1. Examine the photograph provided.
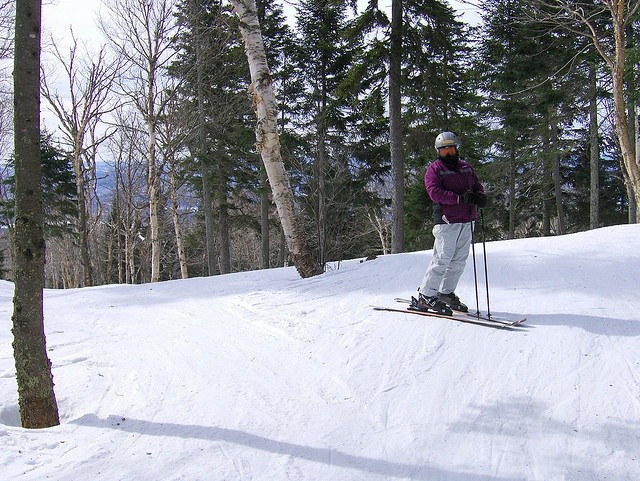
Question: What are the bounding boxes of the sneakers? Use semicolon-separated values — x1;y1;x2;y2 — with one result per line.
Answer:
437;292;467;312
418;294;452;316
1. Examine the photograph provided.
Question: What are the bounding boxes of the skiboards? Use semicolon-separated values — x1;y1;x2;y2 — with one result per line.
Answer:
373;298;526;328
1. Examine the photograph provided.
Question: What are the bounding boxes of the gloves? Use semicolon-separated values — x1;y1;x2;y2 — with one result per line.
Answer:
475;193;487;208
460;190;475;206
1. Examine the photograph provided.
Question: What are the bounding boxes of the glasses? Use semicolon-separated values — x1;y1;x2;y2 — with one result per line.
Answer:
439;146;457;156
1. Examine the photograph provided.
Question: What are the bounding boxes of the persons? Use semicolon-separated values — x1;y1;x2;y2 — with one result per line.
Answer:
417;132;487;316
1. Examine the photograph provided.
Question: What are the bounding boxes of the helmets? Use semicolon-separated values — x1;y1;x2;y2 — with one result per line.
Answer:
434;131;460;155
